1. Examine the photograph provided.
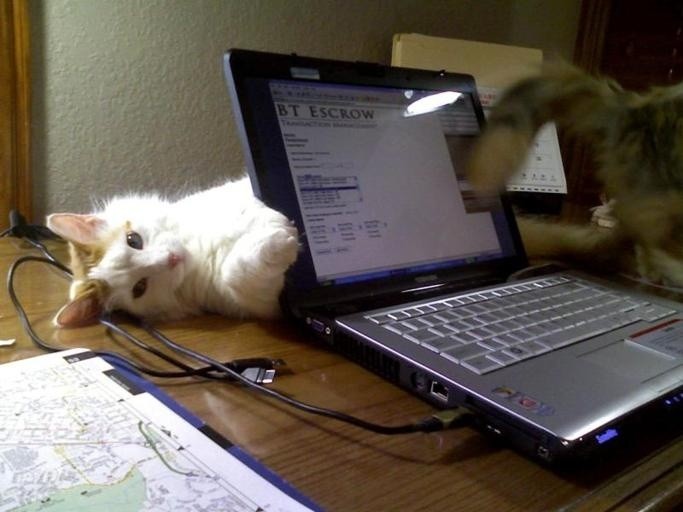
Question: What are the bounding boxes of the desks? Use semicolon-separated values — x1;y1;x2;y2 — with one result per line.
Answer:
0;224;683;512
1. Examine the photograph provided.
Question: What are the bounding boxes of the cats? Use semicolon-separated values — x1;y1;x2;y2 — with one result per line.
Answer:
466;66;683;288
45;172;614;329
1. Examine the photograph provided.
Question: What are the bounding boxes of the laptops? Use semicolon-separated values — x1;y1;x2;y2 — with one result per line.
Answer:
224;47;683;465
